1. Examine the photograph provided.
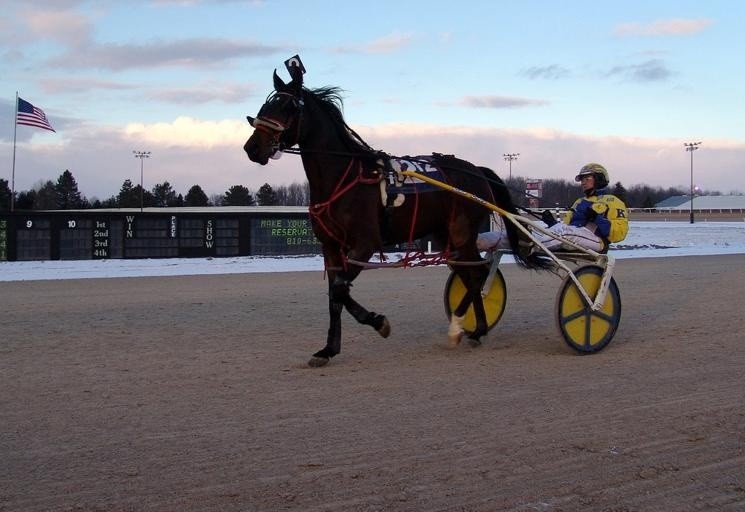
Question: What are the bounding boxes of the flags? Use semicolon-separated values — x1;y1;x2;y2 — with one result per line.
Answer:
16;97;56;133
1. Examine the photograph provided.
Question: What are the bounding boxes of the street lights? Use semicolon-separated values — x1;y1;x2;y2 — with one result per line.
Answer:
501;151;520;204
132;148;152;209
682;139;704;225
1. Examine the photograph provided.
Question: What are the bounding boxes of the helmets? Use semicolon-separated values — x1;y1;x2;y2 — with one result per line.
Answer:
574;161;609;183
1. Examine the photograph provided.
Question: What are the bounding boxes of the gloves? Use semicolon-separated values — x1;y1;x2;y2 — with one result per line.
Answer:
542;209;554;224
584;207;597;221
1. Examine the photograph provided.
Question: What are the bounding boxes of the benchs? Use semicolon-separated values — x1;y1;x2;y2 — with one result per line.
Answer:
493;248;607;257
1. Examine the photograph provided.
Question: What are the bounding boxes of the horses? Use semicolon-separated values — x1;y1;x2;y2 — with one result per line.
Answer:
243;65;566;368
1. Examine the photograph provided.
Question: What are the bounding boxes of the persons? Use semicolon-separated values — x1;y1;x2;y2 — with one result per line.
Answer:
476;162;630;258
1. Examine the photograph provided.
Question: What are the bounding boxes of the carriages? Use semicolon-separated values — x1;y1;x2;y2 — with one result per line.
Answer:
238;62;624;370
